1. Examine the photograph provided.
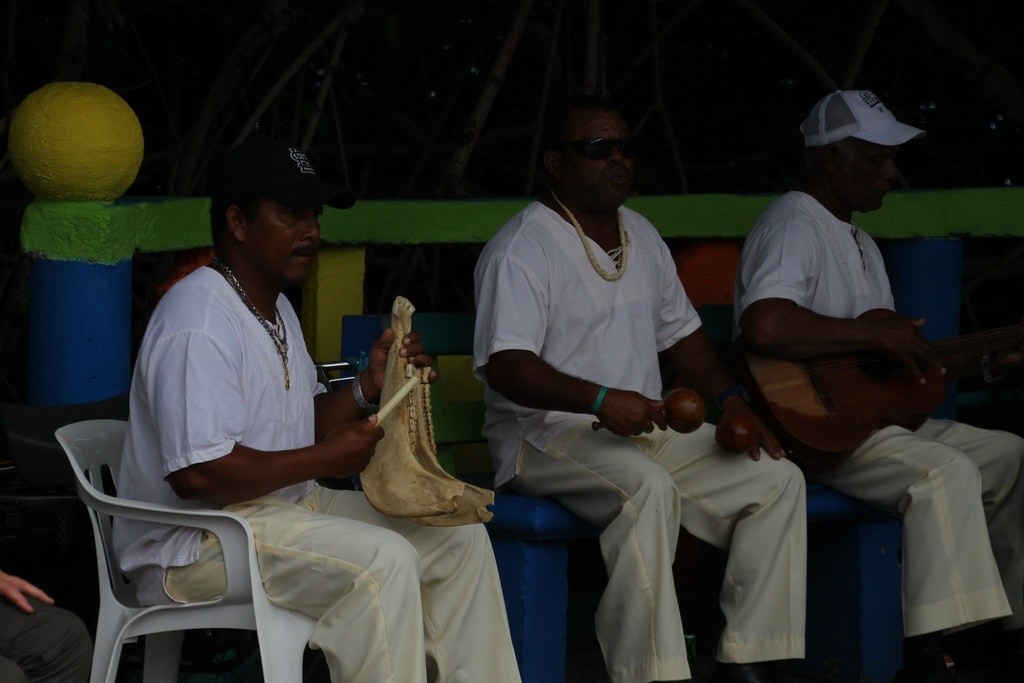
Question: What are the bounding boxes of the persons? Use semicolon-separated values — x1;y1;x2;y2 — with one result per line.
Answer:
473;97;806;683
112;139;523;683
732;91;1024;683
0;569;94;683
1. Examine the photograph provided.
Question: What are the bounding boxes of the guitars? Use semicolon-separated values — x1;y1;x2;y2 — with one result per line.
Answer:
732;298;1024;470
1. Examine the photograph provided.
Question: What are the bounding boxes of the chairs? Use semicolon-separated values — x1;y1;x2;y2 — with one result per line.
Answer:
54;418;320;683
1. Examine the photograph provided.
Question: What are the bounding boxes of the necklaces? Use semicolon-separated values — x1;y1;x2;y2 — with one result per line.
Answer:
210;253;291;395
549;186;627;280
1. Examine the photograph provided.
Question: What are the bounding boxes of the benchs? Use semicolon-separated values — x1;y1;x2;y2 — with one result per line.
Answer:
456;471;906;683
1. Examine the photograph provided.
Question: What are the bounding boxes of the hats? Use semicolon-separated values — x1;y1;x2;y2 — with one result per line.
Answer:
801;90;926;147
210;139;327;210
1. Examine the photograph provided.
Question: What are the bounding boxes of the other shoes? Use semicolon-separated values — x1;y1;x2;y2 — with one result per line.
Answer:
709;661;785;683
892;636;1024;683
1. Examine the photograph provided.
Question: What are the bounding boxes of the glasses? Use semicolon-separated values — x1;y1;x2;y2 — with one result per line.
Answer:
546;136;635;160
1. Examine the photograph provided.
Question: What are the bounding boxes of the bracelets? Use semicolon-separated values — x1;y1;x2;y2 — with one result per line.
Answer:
352;373;380;413
591;386;608;416
714;388;742;410
